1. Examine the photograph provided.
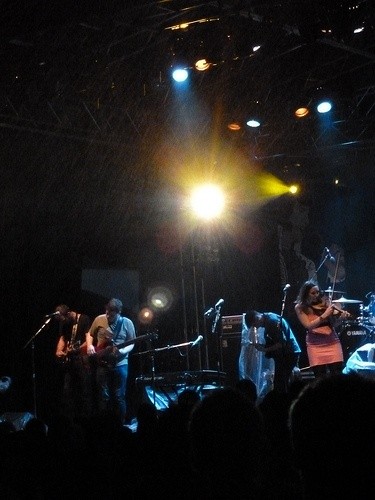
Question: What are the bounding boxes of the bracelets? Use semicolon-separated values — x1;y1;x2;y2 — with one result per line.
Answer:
268;344;273;352
319;316;326;321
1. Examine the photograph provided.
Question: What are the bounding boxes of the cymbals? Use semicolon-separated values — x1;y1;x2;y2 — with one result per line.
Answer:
320;290;344;293
331;299;365;305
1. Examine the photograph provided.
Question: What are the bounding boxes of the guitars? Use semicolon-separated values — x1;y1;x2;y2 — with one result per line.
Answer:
60;341;80;368
238;339;276;360
78;330;159;370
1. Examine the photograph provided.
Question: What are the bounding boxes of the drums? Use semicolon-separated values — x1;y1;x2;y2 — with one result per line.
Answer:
341;320;368;358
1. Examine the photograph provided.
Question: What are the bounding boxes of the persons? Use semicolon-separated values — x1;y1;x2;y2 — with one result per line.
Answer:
322;243;349;310
53;298;136;438
294;280;351;380
245;309;301;393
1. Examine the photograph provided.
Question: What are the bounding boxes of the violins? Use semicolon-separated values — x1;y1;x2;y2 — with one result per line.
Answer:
313;294;353;318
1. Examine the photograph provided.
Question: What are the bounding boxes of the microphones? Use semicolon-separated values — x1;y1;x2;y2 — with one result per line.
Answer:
214;299;224;307
45;311;61;318
204;307;214;316
283;284;291;291
325;246;337;264
191;335;203;347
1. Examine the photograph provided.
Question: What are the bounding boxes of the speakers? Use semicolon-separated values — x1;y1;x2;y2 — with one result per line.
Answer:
219;332;242;375
135;375;195;401
339;324;368;358
